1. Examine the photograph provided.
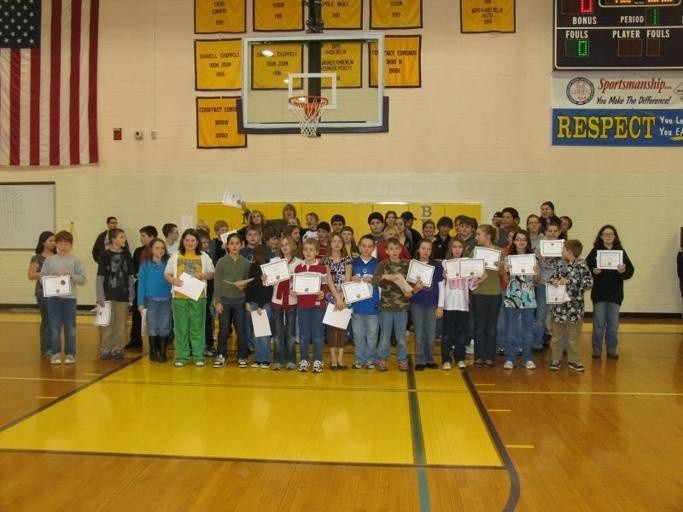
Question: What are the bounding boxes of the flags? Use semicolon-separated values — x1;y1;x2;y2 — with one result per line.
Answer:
0;0;100;167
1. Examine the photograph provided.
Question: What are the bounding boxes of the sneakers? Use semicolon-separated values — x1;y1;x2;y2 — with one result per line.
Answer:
607;353;619;359
102;352;113;359
503;361;512;369
549;360;560;370
45;350;76;363
526;360;536;369
568;361;584;370
115;350;123;359
399;360;408;370
415;359;493;371
592;352;601;357
175;348;323;372
354;361;386;371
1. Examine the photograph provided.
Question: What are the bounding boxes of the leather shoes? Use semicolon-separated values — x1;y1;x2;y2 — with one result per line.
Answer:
330;361;346;369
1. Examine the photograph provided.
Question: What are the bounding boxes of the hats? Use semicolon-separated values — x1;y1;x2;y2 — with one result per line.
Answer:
401;212;417;219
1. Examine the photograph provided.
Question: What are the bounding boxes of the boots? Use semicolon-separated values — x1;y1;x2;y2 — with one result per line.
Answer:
149;337;167;362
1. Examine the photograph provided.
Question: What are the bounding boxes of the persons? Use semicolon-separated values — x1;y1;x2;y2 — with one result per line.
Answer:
585;225;634;360
92;202;593;372
28;231;57;358
39;231;87;365
